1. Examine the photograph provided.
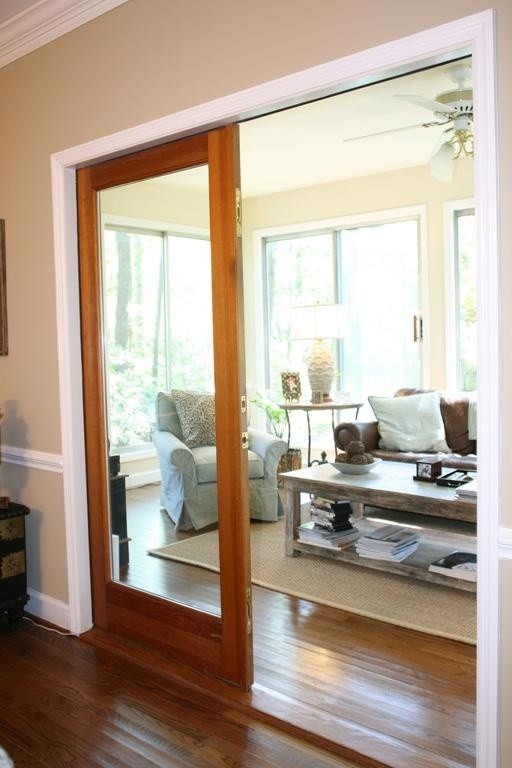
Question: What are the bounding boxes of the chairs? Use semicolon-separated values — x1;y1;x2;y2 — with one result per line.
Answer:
153;389;287;532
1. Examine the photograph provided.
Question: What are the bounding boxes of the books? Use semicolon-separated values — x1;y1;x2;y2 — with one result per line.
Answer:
455;479;478;498
428;551;477;582
295;495;419;563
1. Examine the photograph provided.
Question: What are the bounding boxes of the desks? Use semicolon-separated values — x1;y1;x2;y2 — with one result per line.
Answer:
110;474;132;565
0;501;30;625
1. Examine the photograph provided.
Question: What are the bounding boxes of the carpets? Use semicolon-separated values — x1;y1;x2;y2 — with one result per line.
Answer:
144;501;477;648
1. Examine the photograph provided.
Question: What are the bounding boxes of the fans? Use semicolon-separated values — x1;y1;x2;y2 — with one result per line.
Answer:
342;64;474;182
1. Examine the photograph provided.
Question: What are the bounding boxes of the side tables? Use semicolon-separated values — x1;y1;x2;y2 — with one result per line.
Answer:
279;400;364;467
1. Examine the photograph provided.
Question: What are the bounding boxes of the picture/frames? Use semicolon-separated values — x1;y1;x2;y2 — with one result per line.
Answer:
282;371;301;403
1;218;9;356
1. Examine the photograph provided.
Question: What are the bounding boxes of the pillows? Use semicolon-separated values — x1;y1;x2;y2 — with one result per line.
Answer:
171;390;217;447
367;393;448;452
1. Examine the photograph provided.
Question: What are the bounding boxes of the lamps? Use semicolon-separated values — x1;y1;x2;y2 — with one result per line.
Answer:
292;300;351;404
430;118;475;182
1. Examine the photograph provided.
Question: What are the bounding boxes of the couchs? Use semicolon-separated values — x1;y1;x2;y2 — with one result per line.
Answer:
333;386;476;472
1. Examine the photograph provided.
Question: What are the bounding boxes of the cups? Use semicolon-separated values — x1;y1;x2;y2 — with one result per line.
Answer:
311;389;324;404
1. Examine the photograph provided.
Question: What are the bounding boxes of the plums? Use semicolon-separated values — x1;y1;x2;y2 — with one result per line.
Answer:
335;440;374;465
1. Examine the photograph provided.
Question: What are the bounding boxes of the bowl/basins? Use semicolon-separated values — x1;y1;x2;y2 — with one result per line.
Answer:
328;457;384;473
